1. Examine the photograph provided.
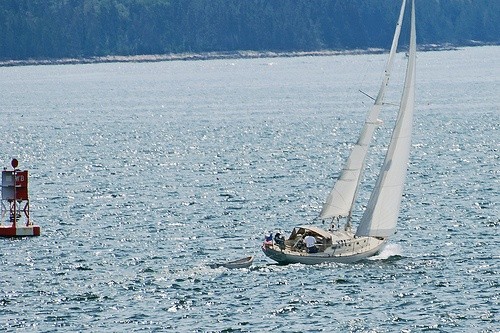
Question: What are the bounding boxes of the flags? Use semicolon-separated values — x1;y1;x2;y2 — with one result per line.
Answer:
264;234;273;246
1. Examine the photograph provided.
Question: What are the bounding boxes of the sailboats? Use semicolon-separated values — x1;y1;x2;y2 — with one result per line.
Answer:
262;0;417;265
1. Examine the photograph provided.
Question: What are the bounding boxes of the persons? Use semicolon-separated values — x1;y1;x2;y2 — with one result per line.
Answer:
303;231;319;253
